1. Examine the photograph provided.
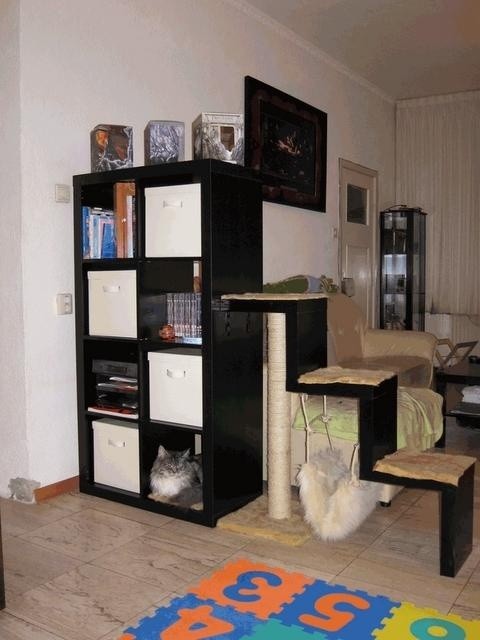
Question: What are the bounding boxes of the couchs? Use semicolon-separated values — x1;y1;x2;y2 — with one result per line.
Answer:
260;288;451;506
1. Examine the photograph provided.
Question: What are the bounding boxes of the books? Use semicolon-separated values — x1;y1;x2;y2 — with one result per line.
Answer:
87;376;139;420
167;261;202;345
82;179;136;259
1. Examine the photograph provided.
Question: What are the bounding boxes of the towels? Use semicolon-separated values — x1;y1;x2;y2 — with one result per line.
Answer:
370;446;478;489
296;362;398;386
217;290;329;302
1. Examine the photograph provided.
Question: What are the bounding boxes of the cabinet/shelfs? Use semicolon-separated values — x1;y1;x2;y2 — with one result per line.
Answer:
71;155;268;529
378;208;427;333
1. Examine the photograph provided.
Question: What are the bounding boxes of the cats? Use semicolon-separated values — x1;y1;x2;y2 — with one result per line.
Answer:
146;443;203;506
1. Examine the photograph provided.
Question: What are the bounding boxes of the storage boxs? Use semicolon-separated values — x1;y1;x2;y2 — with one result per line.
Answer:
142;183;203;260
192;111;247;166
86;270;140;340
147;345;206;430
92;414;141;495
88;124;134;173
144;118;187;165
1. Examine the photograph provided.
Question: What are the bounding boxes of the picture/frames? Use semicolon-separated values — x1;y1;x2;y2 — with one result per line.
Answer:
242;76;329;214
